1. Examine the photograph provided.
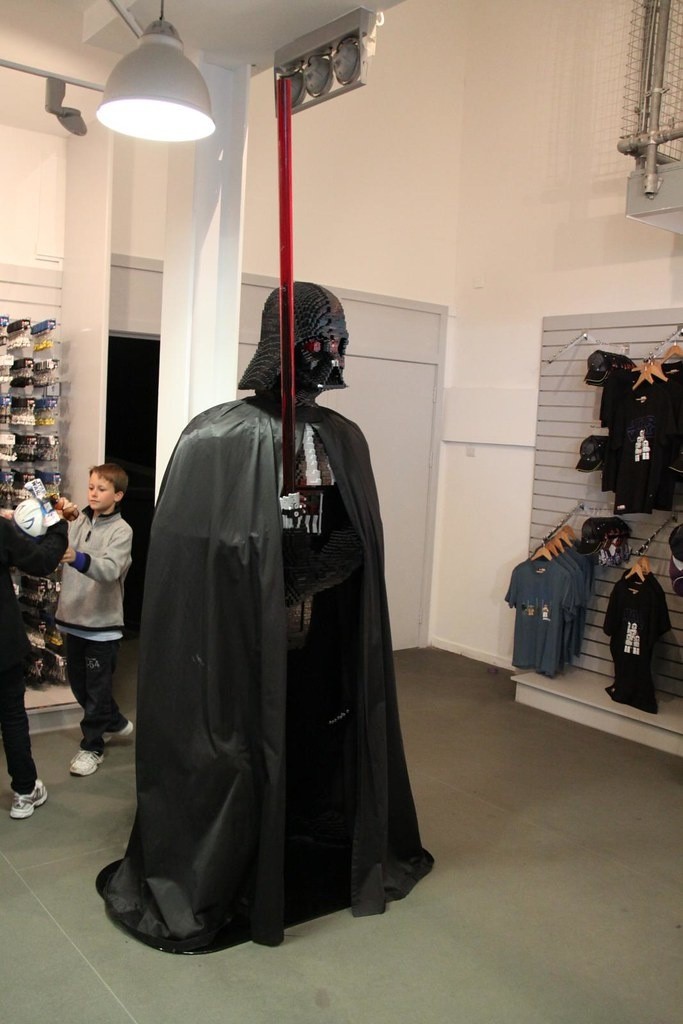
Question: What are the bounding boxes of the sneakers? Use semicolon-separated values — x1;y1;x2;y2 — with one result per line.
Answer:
10;779;48;818
69;749;104;776
101;720;133;741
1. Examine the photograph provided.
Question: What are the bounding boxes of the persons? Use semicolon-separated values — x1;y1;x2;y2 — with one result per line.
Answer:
61;465;134;775
96;283;435;956
0;498;69;819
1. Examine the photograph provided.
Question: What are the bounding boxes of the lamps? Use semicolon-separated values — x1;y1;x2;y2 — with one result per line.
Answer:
274;7;385;118
96;0;217;143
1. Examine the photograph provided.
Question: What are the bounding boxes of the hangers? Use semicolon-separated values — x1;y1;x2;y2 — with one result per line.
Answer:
632;334;683;401
625;552;645;593
637;544;651;575
531;517;576;573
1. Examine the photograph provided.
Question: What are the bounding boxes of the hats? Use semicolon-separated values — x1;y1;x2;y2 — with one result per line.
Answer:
584;350;637;387
668;523;683;571
575;435;608;473
574;517;632;555
